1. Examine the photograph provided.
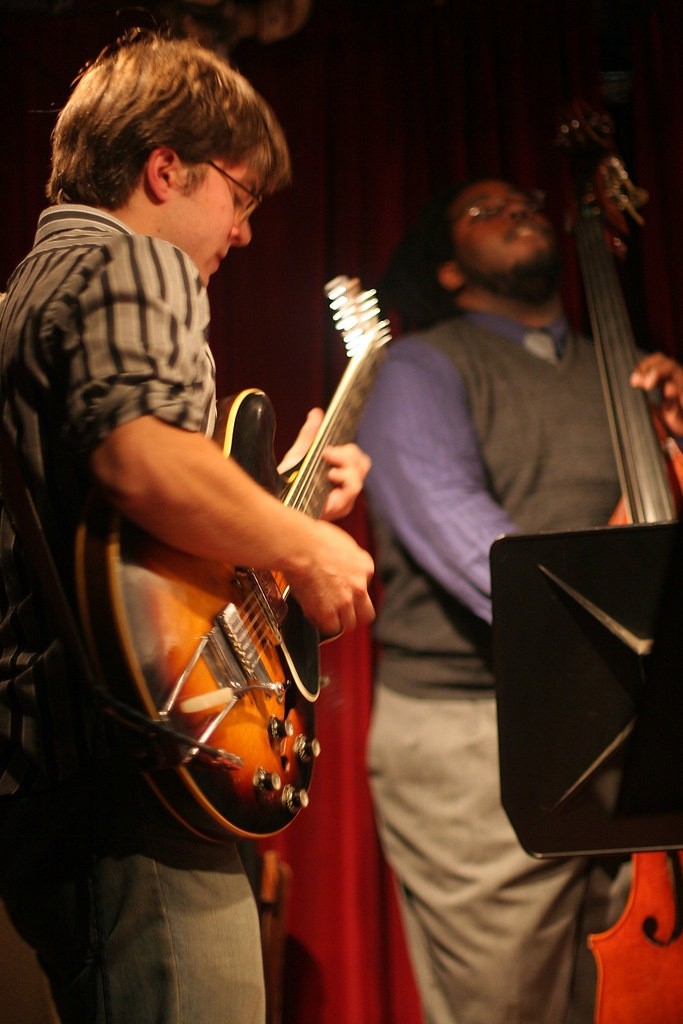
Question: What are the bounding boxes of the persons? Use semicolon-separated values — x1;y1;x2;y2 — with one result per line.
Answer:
0;27;375;1024
355;176;683;1024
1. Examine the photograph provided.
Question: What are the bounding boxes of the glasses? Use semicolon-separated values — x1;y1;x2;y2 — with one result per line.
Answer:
206;159;262;223
450;195;539;251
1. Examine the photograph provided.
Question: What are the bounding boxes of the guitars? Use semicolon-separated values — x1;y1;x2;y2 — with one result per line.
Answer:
77;271;393;848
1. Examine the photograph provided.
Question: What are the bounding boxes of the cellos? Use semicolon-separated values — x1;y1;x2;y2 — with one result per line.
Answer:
557;117;683;1024
